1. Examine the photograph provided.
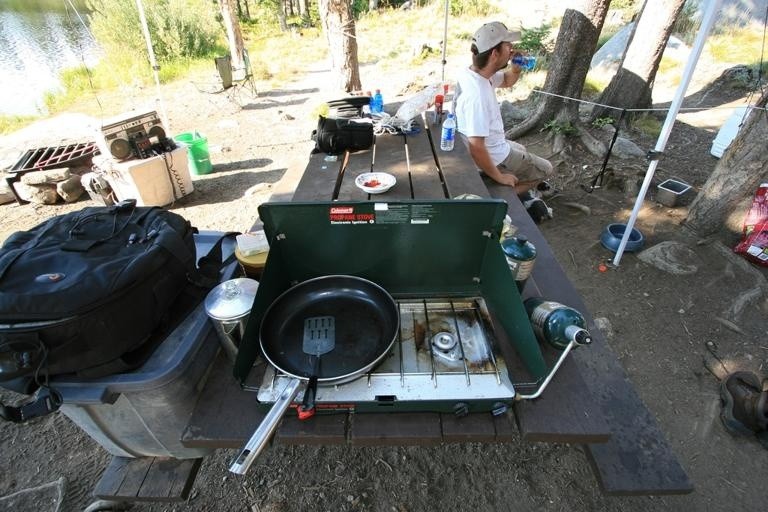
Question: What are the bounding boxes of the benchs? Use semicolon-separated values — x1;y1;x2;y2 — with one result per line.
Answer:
481;175;697;496
90;456;203;504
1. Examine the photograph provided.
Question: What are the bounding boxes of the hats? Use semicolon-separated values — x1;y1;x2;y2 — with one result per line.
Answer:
471;21;522;53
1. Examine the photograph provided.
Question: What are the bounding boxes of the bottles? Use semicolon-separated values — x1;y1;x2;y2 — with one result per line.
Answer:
441;113;455;151
366;89;384;115
443;84;449;102
509;54;536;71
434;95;443;124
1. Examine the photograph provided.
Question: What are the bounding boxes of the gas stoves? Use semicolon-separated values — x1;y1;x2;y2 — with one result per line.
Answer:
253;292;517;411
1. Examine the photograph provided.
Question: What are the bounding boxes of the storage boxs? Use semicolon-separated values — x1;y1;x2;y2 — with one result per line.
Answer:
90;139;194;208
31;226;245;463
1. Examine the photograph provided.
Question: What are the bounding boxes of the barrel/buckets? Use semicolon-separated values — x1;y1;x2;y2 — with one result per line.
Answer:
177;133;213;175
234;245;269;279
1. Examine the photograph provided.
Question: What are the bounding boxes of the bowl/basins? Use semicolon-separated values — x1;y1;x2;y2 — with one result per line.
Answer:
599;222;643;252
355;171;397;194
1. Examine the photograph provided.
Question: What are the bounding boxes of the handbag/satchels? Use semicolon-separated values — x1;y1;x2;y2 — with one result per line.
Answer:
311;114;373;156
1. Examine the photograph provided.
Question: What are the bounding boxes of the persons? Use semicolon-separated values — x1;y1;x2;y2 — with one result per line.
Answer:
449;21;554;195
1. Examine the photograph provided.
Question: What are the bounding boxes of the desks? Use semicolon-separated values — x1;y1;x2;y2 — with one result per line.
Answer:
177;102;615;451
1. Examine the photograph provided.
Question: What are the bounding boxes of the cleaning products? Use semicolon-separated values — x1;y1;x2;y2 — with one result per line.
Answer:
375;90;383;111
367;92;374;113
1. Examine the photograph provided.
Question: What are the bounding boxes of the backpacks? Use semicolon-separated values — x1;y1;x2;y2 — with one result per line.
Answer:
0;199;222;423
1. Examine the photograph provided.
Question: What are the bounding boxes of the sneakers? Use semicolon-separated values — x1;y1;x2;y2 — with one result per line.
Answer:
720;370;768;437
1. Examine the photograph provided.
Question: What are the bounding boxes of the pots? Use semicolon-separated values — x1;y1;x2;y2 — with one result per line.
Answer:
227;275;399;478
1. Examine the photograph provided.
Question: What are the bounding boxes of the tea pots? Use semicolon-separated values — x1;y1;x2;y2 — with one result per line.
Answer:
203;277;261;369
